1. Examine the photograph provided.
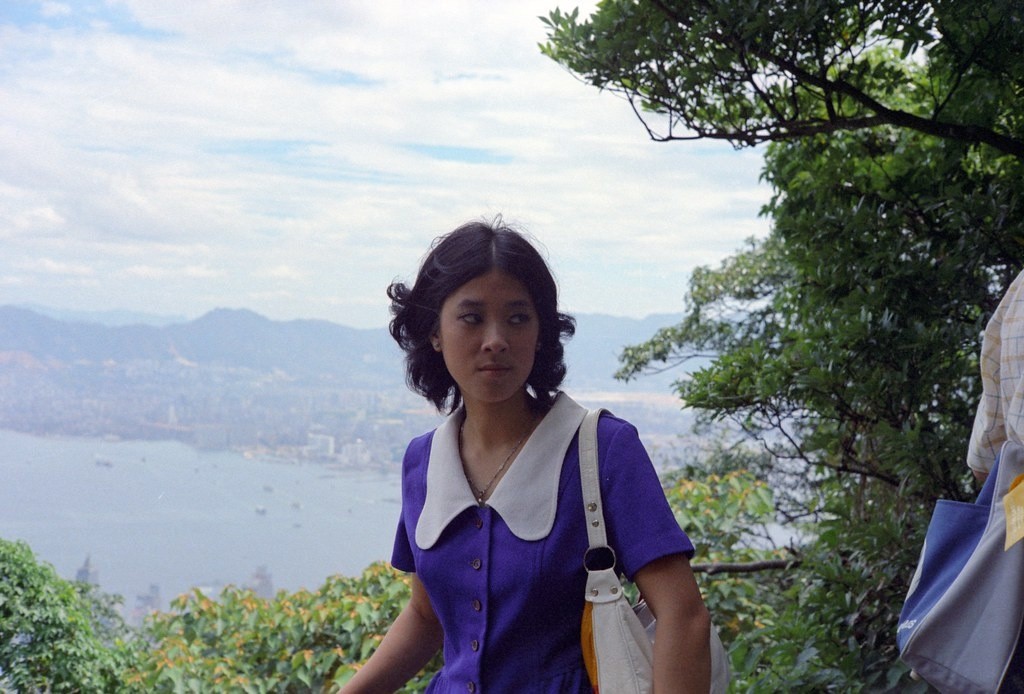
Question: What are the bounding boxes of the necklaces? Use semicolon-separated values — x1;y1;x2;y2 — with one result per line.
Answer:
457;419;537;501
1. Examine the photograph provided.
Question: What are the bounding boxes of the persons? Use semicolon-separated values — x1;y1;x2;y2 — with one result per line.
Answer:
891;266;1024;694
330;219;714;694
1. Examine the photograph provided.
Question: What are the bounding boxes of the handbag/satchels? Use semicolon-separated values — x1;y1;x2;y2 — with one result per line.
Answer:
579;409;731;694
896;439;1024;694
